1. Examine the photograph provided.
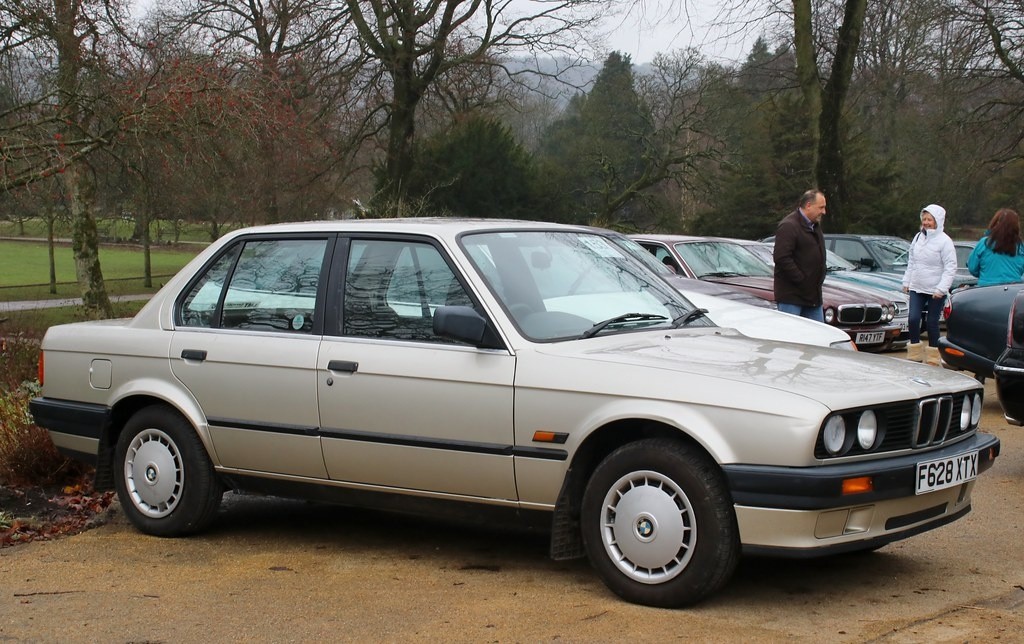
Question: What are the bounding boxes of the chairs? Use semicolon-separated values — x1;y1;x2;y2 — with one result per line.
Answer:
447;274;509;307
345;271;400;339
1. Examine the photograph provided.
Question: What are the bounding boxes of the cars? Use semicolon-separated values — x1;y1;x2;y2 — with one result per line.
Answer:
625;233;904;345
186;236;860;350
755;234;982;294
765;242;950;334
937;279;1024;379
26;212;1002;610
993;282;1024;428
577;224;777;309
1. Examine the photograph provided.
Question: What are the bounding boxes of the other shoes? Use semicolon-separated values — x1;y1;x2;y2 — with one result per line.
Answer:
973;374;985;384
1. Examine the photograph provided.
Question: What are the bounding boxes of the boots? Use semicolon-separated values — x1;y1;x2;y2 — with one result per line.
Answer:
906;340;923;363
924;346;941;366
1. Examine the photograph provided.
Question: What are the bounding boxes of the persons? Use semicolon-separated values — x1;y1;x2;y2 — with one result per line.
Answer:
966;207;1024;384
902;203;959;369
771;188;827;325
